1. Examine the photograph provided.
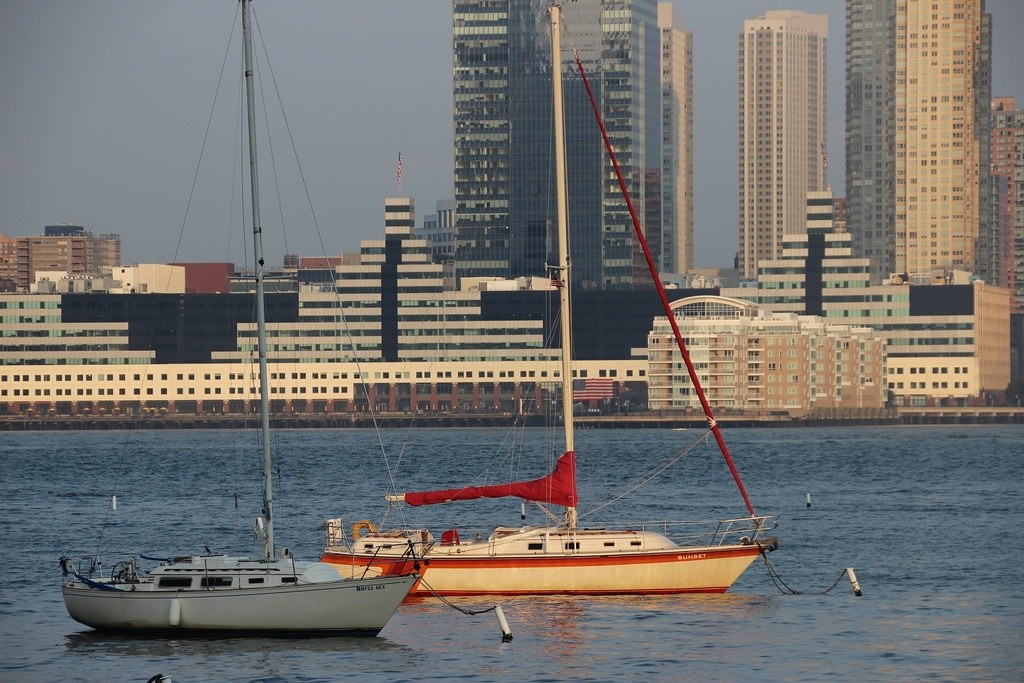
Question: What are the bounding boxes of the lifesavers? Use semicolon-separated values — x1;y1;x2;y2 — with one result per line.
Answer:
351;520;377;541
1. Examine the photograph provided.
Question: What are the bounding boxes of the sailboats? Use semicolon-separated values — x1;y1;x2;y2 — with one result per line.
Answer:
59;0;418;636
319;0;778;600
